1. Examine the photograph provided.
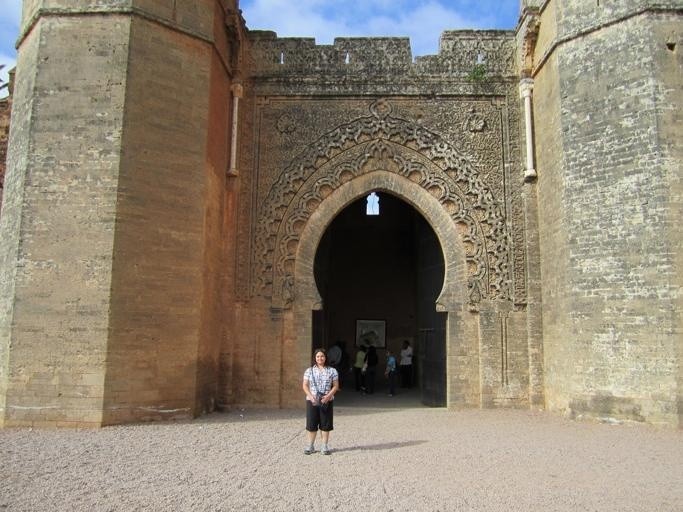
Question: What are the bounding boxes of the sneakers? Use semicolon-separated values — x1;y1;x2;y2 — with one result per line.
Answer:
304;446;315;455
320;446;329;456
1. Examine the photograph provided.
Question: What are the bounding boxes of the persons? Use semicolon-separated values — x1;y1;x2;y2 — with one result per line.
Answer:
326;343;342;369
302;347;339;455
353;344;366;391
360;345;378;394
383;349;397;397
398;340;413;393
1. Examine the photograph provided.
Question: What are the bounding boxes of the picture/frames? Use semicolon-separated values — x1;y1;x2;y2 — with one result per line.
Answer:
355;318;387;349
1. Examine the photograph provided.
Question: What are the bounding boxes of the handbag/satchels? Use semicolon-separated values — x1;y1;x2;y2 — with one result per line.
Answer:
314;392;329;406
